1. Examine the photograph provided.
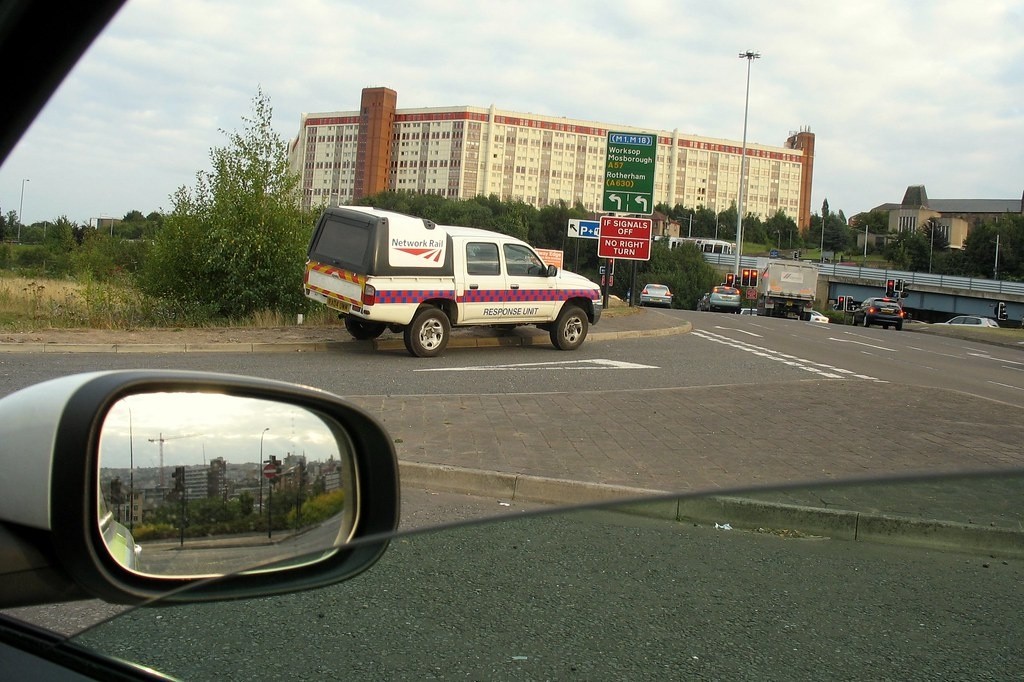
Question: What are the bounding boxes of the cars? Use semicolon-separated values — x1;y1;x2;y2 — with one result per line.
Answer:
697;285;757;315
934;315;1001;329
850;296;903;331
640;283;674;309
810;310;829;323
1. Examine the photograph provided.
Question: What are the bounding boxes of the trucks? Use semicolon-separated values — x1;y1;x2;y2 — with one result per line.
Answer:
757;260;817;320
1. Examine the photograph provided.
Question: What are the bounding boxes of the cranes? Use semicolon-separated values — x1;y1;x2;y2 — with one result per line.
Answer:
148;432;205;487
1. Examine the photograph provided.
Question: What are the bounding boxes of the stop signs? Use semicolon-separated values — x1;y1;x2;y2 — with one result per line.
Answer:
263;464;277;479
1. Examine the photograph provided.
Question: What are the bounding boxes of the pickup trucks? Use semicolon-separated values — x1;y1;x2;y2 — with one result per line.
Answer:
303;203;603;359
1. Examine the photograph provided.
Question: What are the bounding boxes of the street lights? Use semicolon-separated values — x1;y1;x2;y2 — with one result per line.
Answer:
989;234;1002;278
733;47;762;276
260;427;270;517
927;219;934;274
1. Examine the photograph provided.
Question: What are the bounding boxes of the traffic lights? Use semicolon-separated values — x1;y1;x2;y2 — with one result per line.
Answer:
749;269;759;288
740;269;750;286
996;302;1008;321
610;258;615;275
833;296;846;311
895;278;903;292
269;455;282;484
845;296;854;311
172;466;184;491
721;273;735;288
900;281;910;299
732;273;742;288
885;279;896;297
792;248;802;259
